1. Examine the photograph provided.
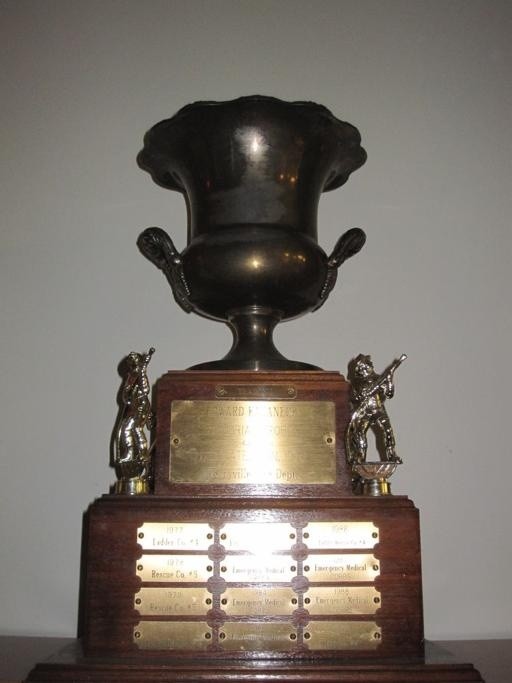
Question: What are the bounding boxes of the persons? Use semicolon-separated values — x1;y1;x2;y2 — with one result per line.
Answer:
346;352;401;464
108;348;158;460
